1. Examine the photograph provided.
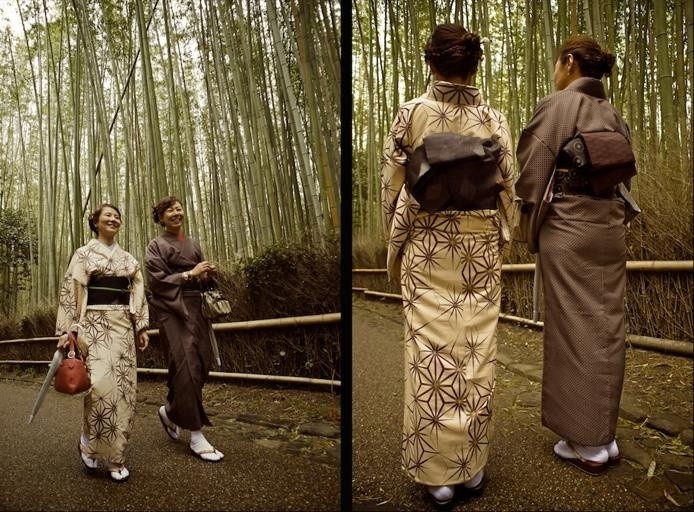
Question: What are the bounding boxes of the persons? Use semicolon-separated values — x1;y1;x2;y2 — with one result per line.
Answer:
515;32;643;478
379;23;522;510
142;197;230;463
52;203;153;483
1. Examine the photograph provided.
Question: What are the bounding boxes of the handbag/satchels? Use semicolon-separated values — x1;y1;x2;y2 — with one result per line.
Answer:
52;358;89;393
202;291;231;319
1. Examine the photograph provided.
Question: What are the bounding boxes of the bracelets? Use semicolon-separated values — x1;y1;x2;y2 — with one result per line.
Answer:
188;270;192;280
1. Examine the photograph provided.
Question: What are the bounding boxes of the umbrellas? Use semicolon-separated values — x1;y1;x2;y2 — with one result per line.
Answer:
532;253;541;323
27;323;84;424
206;319;221;366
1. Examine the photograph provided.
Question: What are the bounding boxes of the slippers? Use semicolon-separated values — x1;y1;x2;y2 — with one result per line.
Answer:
434;494;455;509
469;478;486;498
78;443;98;471
190;444;224;463
158;406;180;441
553;438;622;475
106;462;129;482
